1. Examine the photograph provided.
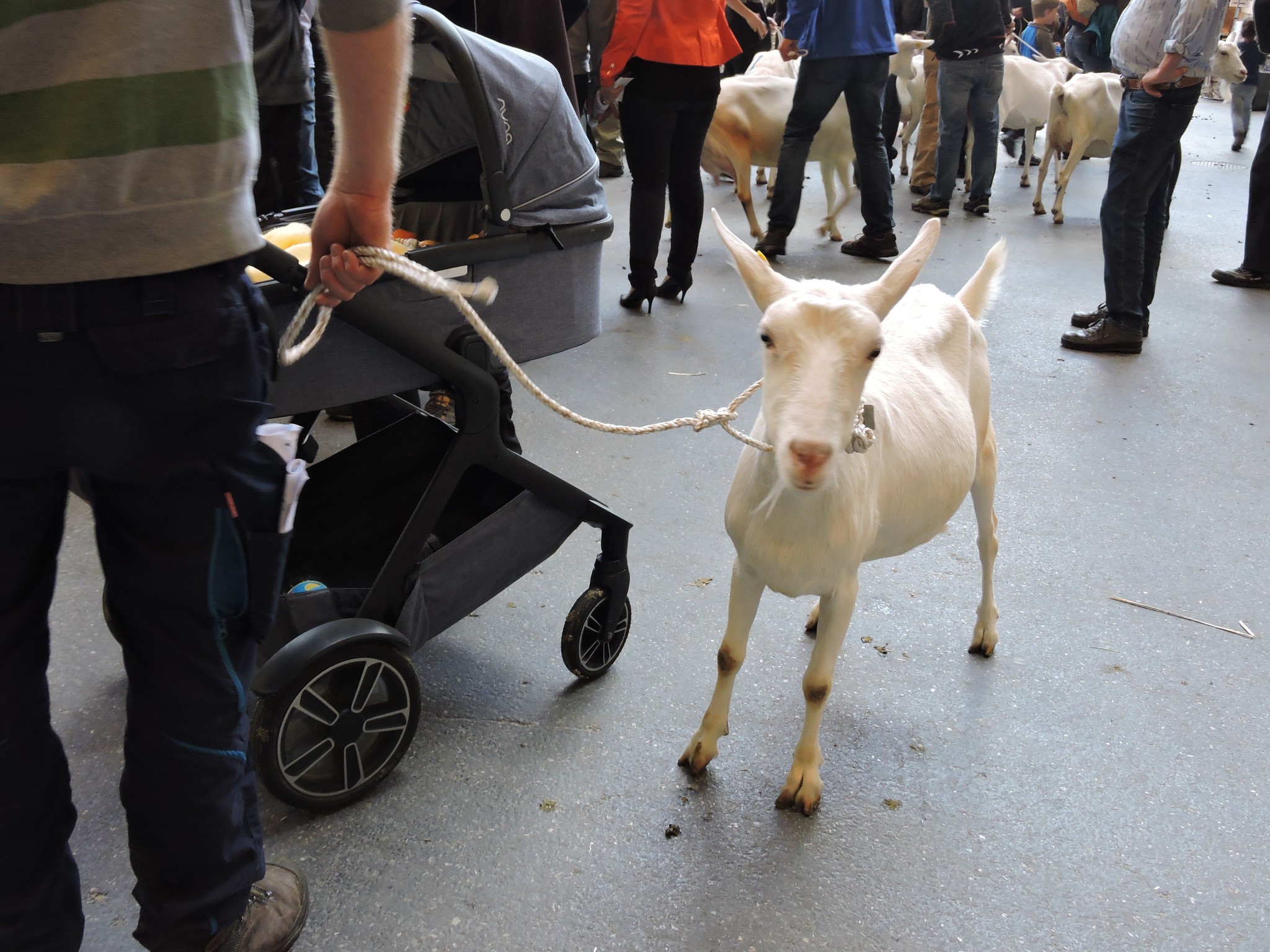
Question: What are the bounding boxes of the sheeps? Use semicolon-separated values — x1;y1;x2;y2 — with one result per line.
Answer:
670;203;1013;822
698;28;1252;242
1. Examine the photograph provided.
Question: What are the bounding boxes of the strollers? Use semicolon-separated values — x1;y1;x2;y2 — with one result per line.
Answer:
235;1;633;810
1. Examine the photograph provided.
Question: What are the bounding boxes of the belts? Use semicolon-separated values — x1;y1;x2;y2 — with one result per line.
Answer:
1117;74;1205;91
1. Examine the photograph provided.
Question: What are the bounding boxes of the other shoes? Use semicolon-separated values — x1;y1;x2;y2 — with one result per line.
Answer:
1060;318;1142;354
1212;267;1270;287
1018;154;1043;166
1001;133;1016;157
911;199;950;217
963;197;990;212
598;160;624;178
1070;302;1149;337
1061;151;1091;160
1232;132;1246;151
910;184;935;193
208;855;310;952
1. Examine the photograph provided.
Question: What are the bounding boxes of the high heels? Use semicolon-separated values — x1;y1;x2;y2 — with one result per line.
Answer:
619;284;656;314
656;271;692;304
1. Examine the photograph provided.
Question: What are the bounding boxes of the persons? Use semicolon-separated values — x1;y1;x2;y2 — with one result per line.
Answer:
558;0;624;179
1062;0;1230;354
717;0;788;182
1211;0;1270;289
1231;17;1267;152
0;0;411;952
852;0;1130;217
600;0;743;314
753;0;899;257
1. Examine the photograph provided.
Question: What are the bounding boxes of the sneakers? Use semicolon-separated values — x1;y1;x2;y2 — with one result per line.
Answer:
754;229;788;256
842;232;898;256
